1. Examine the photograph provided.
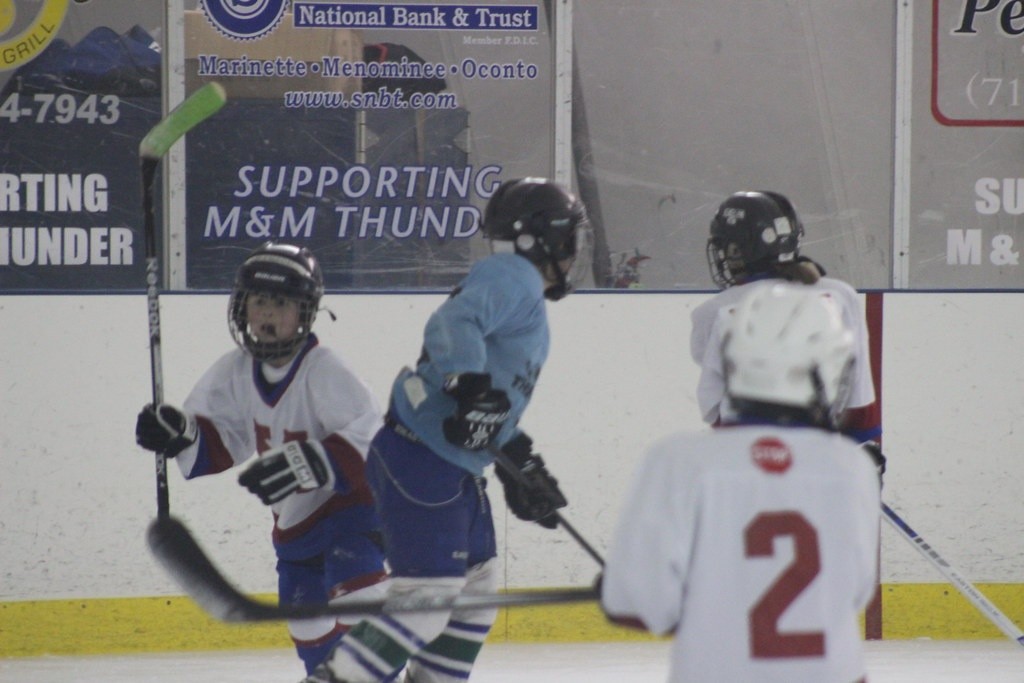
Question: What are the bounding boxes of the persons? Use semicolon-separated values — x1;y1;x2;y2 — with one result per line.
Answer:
590;284;883;683
688;189;875;440
135;241;383;681
297;175;589;683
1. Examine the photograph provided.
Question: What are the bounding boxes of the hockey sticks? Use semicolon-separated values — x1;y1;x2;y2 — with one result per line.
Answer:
133;78;608;625
879;504;1024;645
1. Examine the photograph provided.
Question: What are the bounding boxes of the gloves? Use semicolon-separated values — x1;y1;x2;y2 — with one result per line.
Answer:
442;372;512;451
495;432;567;530
134;403;199;459
238;440;328;506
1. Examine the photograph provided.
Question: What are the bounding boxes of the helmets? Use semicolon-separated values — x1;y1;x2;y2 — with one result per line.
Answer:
482;177;595;302
719;283;861;429
227;240;337;363
706;190;805;290
